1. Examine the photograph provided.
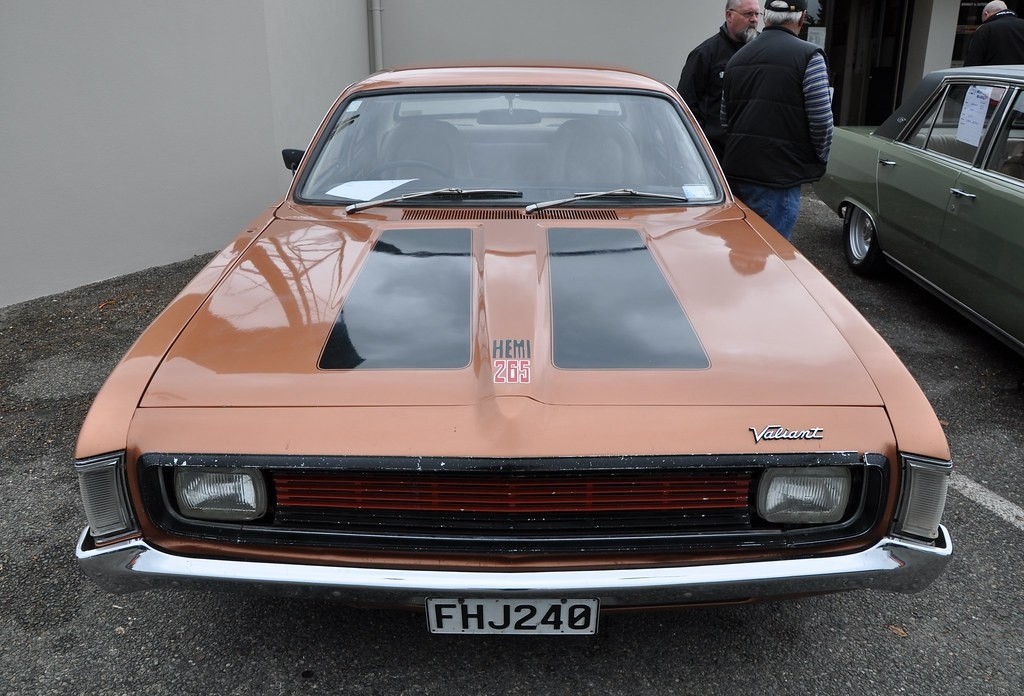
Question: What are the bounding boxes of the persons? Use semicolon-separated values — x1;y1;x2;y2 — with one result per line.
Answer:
676;0;761;165
962;0;1024;119
721;0;834;241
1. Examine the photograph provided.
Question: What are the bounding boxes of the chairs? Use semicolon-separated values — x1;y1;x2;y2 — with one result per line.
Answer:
549;118;643;186
378;120;469;188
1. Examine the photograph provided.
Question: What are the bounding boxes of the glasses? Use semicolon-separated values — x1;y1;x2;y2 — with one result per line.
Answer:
729;8;763;19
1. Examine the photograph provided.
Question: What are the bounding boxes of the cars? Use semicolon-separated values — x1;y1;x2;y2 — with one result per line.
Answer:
816;64;1024;360
74;65;954;637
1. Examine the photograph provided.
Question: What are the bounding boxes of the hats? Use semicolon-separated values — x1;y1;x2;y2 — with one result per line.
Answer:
764;0;807;13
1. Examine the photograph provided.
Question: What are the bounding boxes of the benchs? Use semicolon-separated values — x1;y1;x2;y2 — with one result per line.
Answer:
918;133;1024;162
